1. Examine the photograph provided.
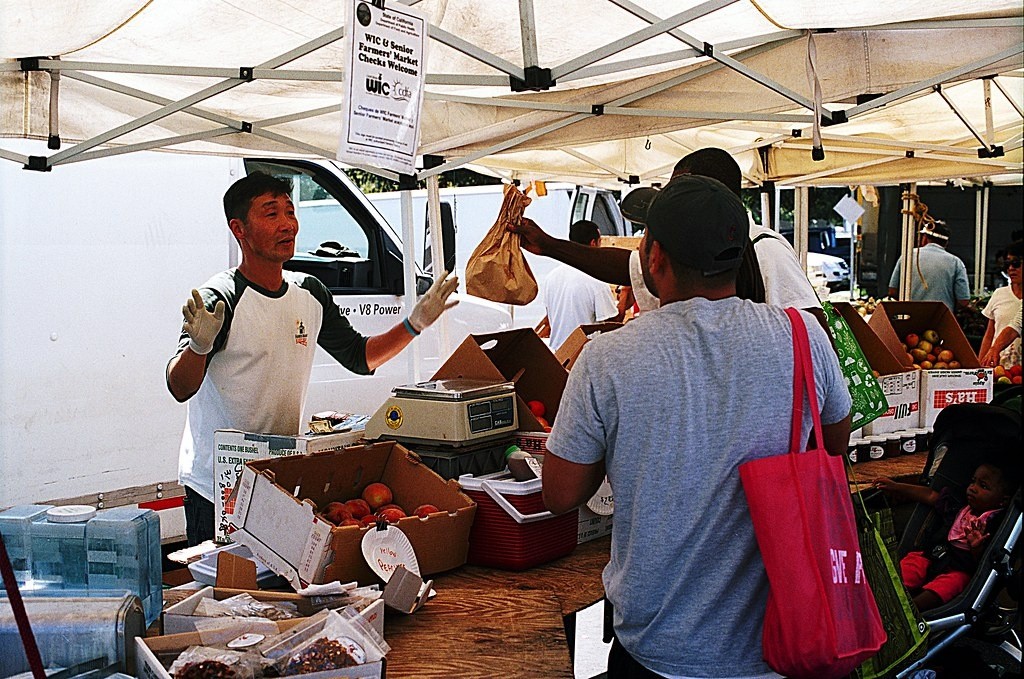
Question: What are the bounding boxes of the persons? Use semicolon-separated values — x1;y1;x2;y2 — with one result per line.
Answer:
889;223;970;311
982;306;1023;367
165;170;461;545
978;227;1024;365
544;220;631;349
506;148;838;360
873;458;1015;611
543;174;853;679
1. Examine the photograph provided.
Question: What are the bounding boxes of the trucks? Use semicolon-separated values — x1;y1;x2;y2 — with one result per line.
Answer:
297;183;633;306
0;137;512;510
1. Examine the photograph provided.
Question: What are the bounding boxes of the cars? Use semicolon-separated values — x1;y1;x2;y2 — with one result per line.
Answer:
807;252;849;286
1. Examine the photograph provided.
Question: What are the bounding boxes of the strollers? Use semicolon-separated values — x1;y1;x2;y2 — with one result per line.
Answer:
888;384;1024;679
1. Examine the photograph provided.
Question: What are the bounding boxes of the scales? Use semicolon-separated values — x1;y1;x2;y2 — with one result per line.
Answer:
364;378;519;448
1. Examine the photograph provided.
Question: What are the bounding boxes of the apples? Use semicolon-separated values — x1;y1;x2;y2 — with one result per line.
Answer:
529;400;552;433
985;364;1022;384
871;370;880;378
902;330;960;368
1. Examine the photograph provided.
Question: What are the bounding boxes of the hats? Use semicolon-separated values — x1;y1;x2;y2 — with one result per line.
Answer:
917;219;950;241
620;174;749;274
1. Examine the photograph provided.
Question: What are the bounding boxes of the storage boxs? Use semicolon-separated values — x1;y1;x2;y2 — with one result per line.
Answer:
832;301;904;376
134;585;387;679
516;430;615;545
429;328;570;433
862;366;921;437
919;367;994;428
458;465;579;570
228;440;477;593
214;411;372;543
553;322;625;372
868;300;980;369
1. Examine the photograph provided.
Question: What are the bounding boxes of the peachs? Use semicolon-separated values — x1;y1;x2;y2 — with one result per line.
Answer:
321;484;439;528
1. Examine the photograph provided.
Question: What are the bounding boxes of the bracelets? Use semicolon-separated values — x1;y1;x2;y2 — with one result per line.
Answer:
403;319;420;336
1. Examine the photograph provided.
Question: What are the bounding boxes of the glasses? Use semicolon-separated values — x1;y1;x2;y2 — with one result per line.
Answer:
1003;258;1024;271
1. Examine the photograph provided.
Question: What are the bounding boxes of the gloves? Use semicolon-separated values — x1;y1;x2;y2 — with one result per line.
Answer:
182;289;225;356
403;270;461;336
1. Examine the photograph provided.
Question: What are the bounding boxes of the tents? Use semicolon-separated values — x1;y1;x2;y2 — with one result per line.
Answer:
0;0;1024;383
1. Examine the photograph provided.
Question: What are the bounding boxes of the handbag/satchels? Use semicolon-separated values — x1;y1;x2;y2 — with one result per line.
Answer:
738;308;889;679
751;233;889;434
842;450;931;679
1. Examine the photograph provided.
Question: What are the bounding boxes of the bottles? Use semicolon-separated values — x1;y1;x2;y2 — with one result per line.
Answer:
505;445;537;481
